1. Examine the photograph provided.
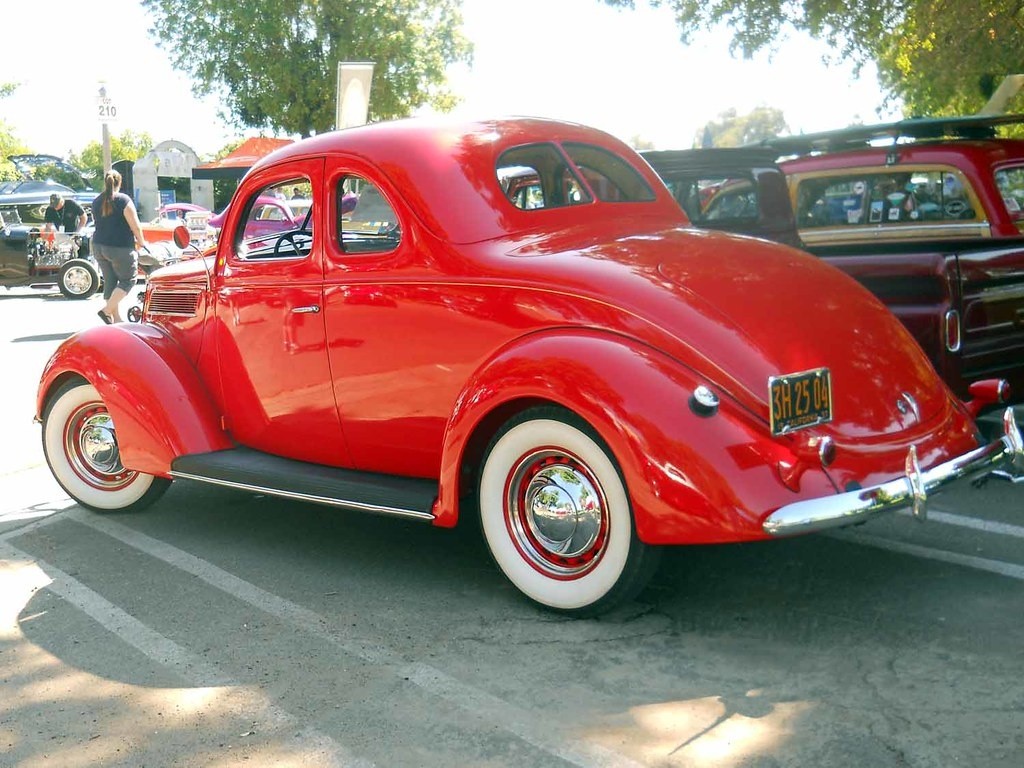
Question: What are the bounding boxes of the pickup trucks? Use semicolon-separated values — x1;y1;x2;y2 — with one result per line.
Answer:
501;146;1022;416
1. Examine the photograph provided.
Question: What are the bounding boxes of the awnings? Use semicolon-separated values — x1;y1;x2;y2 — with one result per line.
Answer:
191;137;295;179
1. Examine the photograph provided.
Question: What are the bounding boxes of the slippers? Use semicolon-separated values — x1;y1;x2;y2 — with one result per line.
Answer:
98;311;112;324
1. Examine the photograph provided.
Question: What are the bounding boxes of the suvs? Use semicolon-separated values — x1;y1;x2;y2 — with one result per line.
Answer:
698;114;1024;249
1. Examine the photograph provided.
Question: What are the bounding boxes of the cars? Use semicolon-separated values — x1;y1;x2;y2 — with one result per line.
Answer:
32;116;1023;618
0;155;358;301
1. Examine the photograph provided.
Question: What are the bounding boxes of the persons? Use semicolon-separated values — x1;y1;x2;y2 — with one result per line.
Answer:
275;187;287;200
291;188;305;199
43;193;88;236
176;210;185;223
91;170;146;325
266;187;275;197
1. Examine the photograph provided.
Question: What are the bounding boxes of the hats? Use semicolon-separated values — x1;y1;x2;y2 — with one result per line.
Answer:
50;194;60;208
